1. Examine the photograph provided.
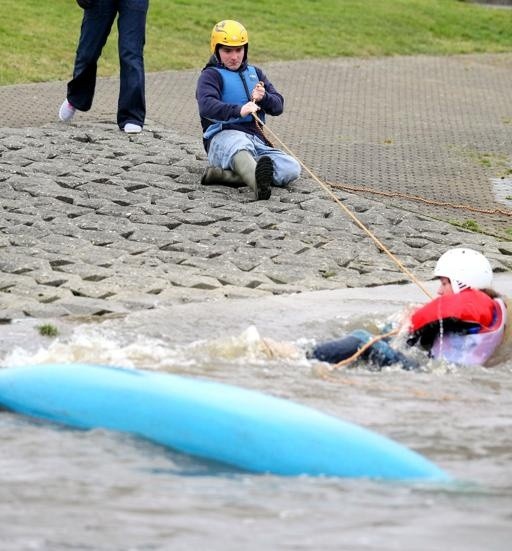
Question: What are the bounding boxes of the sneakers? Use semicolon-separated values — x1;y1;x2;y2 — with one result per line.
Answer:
124;124;142;134
58;97;74;120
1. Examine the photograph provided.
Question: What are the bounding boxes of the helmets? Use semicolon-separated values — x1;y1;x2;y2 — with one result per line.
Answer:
429;248;493;295
211;19;249;56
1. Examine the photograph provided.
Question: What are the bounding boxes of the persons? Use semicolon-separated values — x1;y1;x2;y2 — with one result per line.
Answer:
247;248;506;372
194;19;302;201
58;0;149;134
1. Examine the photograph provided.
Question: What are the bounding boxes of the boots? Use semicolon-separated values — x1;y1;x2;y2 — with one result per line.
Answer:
201;166;246;187
233;149;273;200
